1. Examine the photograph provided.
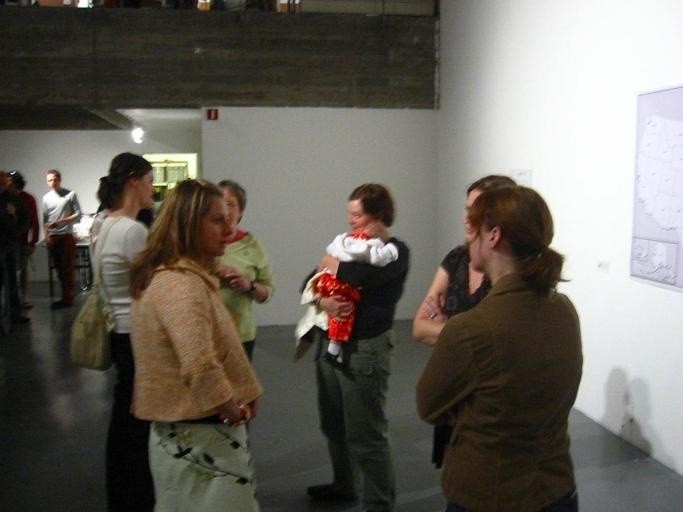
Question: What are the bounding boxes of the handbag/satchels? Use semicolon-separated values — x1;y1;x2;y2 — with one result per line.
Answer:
66;285;113;371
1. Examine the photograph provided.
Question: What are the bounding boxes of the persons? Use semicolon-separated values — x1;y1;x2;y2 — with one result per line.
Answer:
1;172;39;324
205;179;276;365
299;184;410;512
412;175;518;470
416;186;583;512
42;170;82;309
127;177;263;511
91;153;157;512
295;221;399;372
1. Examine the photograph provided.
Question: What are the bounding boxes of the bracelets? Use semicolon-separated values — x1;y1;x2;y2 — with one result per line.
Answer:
245;280;256;296
314;296;322;311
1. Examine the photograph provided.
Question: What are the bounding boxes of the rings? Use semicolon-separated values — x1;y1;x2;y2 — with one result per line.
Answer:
431;313;436;319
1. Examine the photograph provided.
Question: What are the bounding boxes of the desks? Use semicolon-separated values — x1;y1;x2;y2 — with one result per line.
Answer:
47;241;90;298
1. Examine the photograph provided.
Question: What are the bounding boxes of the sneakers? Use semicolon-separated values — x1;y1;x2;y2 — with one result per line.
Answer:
307;483;360;502
0;298;34;325
51;299;73;310
